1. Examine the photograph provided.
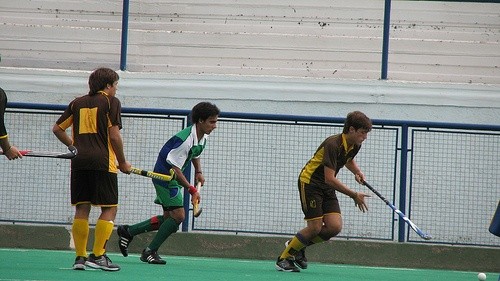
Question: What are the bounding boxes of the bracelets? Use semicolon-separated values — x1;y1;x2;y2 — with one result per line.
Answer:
195;171;202;174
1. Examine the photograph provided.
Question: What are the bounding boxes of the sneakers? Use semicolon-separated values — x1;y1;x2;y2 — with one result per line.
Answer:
285;239;308;269
73;256;87;270
117;225;134;257
84;254;120;271
275;256;301;272
140;248;167;264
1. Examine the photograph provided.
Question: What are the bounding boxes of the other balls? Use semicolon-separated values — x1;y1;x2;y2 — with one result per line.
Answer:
477;272;487;281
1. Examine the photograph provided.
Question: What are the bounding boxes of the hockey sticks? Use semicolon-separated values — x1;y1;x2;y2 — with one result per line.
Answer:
117;165;177;182
0;145;78;159
360;177;433;240
193;180;203;217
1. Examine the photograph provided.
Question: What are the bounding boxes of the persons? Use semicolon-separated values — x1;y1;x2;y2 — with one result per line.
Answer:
0;88;22;160
117;101;220;264
274;110;372;272
52;67;131;271
